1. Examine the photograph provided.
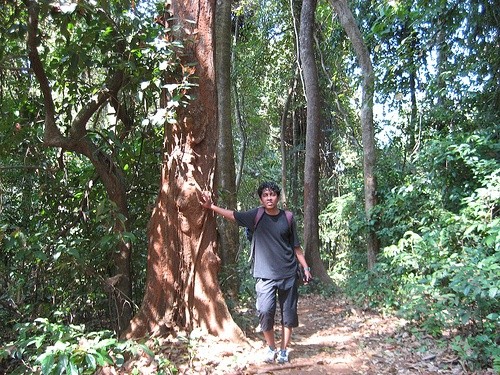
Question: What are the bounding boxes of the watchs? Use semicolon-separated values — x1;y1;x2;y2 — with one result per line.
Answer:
303;267;311;271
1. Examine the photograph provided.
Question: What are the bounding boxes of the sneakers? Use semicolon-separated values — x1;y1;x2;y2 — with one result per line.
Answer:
276;348;290;364
262;347;278;363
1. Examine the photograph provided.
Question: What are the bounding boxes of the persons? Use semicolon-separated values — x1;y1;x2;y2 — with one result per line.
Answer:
199;182;314;363
